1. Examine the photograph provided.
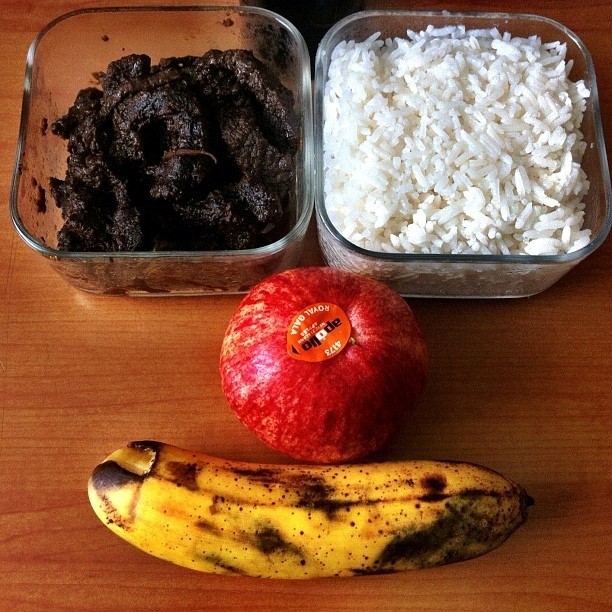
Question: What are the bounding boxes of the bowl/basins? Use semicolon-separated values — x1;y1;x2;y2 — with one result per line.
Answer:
9;1;313;298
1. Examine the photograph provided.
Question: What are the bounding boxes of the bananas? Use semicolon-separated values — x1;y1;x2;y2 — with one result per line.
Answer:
88;441;534;578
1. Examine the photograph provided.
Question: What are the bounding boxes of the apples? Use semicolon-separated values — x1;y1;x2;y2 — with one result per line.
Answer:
221;266;425;463
